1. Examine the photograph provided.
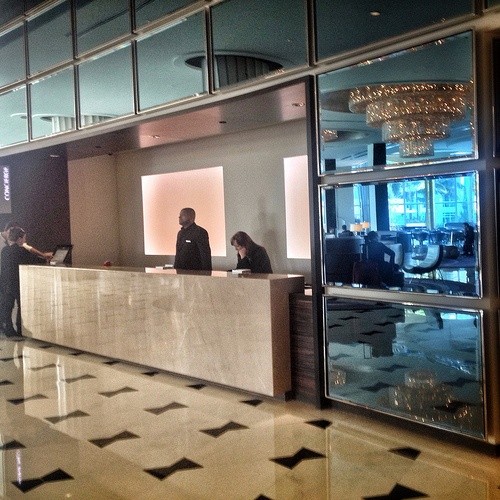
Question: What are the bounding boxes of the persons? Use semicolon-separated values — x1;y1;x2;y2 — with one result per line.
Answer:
0;222;54;336
462;223;474;255
174;208;213;271
230;231;274;274
340;226;401;272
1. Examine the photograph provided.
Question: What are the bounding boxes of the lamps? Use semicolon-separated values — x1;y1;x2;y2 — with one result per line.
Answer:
349;223;362;236
348;83;470;158
360;221;370;235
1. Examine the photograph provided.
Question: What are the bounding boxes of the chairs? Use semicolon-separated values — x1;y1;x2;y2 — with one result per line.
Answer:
328;229;467;287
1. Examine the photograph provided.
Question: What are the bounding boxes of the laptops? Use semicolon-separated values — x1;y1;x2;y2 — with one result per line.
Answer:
48;244;74;264
